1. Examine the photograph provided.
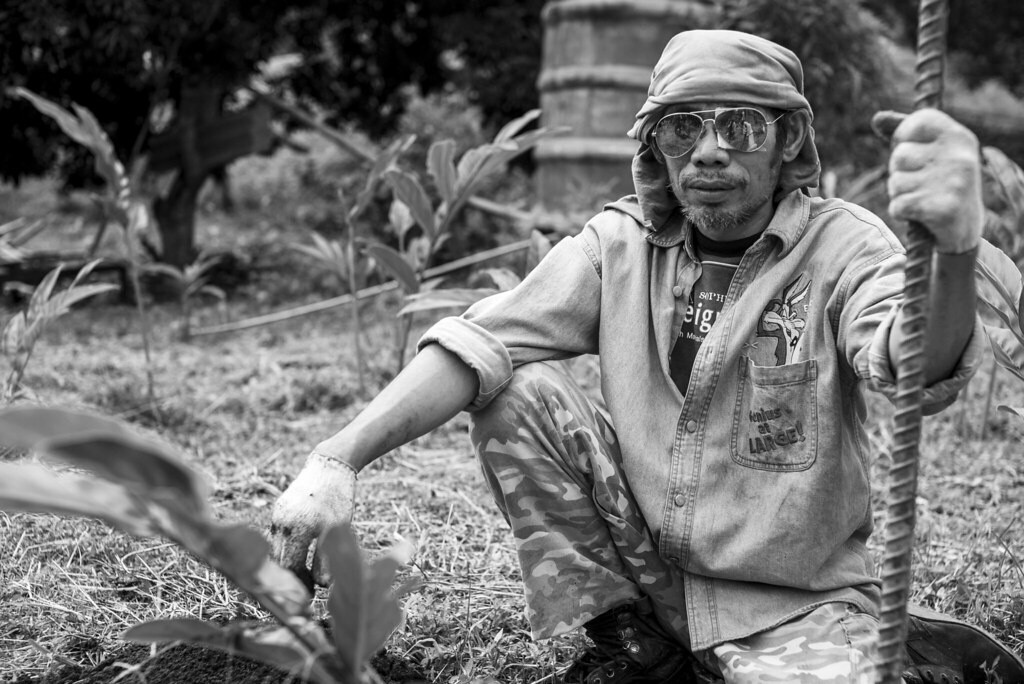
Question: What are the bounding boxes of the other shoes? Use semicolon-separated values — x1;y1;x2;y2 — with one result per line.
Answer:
561;604;699;684
903;598;1024;683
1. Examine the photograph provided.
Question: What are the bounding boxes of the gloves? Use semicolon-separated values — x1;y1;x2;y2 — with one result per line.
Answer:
269;451;357;598
873;108;985;252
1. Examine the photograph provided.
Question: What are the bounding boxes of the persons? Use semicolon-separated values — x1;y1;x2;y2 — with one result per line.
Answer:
274;30;988;684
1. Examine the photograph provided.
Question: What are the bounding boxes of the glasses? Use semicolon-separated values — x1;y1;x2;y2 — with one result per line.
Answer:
651;108;787;159
